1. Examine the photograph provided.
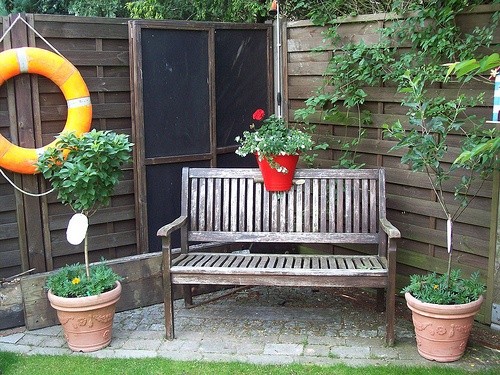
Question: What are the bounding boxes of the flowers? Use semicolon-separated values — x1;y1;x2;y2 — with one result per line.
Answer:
380;51;500;305
234;109;320;174
31;127;136;299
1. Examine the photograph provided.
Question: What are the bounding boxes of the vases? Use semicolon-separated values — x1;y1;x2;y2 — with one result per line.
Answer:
404;291;485;364
46;280;123;354
253;151;300;192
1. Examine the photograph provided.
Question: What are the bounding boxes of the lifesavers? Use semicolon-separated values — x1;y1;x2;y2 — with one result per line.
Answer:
0;47;93;175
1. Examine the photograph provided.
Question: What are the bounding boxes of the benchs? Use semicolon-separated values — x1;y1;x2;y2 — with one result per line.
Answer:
154;166;401;348
